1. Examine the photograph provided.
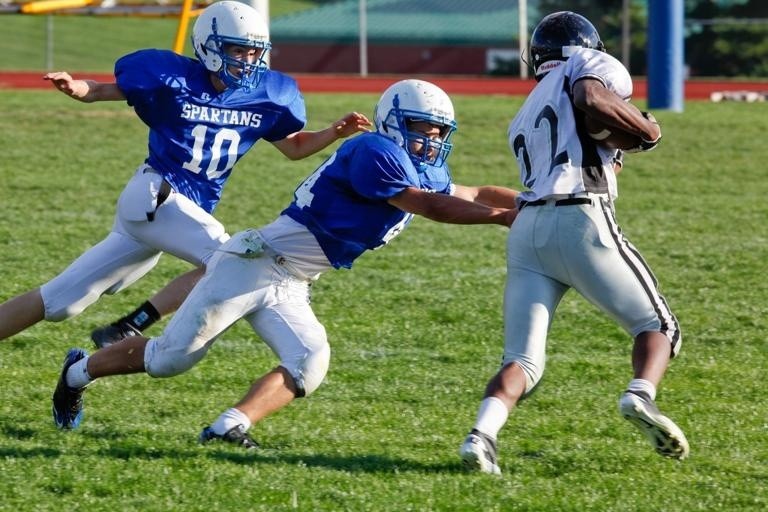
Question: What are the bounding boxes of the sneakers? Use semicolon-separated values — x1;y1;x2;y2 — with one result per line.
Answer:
619;389;690;460
460;428;500;474
52;348;88;431
92;323;127;348
199;424;259;450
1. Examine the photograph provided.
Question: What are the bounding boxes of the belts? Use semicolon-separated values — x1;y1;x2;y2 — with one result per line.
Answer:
518;197;592;211
250;229;317;284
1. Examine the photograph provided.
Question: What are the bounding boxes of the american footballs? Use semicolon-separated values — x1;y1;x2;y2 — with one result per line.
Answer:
582;111;659;152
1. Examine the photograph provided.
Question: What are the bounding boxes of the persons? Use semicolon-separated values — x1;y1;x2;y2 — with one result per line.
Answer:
52;78;521;450
456;11;690;479
2;0;372;351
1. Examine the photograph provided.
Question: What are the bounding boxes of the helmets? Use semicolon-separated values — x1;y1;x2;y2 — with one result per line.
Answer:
373;78;457;168
191;1;273;93
529;11;605;82
